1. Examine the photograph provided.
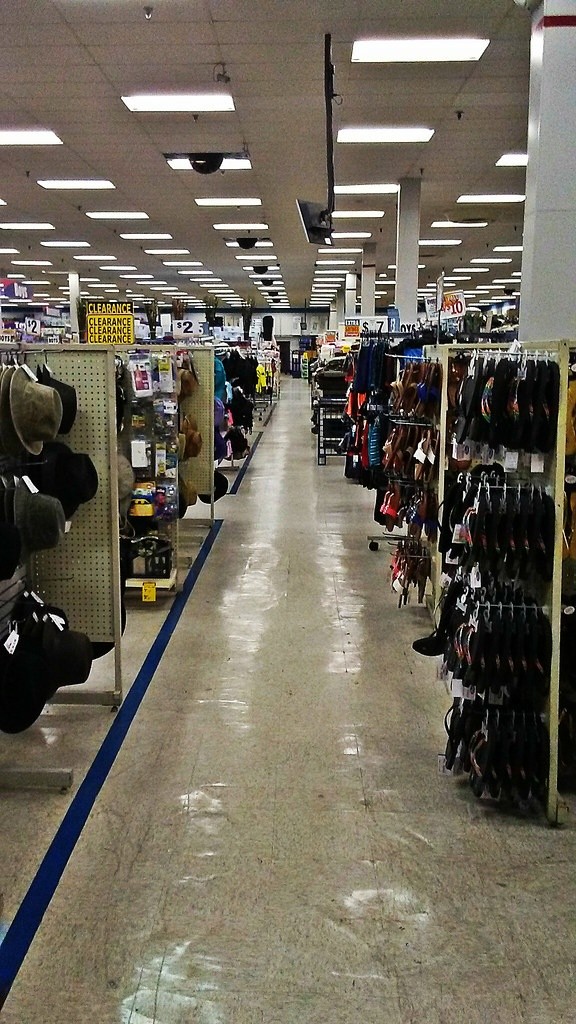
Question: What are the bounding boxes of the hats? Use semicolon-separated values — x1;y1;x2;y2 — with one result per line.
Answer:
175;368;202;519
0;361;93;733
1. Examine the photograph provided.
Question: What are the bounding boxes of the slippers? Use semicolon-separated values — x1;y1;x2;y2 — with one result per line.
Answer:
381;355;442;610
412;354;561;809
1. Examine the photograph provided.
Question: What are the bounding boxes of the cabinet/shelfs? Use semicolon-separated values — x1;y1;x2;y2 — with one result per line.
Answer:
301;358;308;378
